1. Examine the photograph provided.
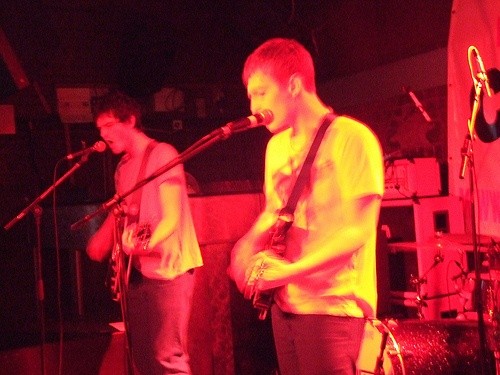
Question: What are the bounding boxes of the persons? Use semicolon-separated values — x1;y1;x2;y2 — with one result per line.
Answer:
93;90;203;374
227;38;385;375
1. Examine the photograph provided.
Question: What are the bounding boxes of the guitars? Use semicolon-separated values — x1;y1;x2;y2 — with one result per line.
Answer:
237;206;294;320
105;222;149;258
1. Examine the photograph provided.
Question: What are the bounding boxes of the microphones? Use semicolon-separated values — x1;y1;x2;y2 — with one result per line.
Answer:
221;110;274;134
473;46;490;98
408;91;431;122
64;141;106;160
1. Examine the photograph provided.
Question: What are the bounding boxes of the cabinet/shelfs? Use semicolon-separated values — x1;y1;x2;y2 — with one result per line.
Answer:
381;197;468;319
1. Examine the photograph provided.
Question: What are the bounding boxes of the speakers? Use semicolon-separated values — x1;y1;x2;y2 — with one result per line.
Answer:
0;206;123;334
